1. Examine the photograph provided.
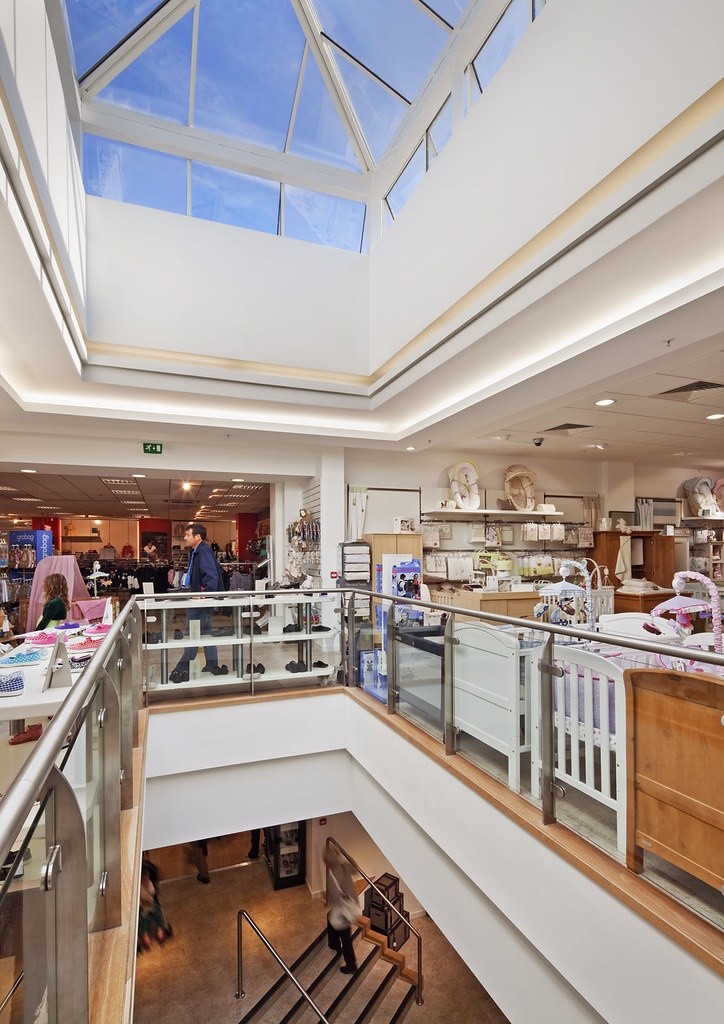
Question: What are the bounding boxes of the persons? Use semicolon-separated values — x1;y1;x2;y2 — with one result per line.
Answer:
322;845;363;974
169;524;225;678
397;574;420;598
137;851;172;957
143;541;156;554
34;574;70;631
396;611;414;627
186;826;273;884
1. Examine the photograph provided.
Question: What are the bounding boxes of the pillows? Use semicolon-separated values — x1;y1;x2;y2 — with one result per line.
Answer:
620;578;655;587
618;587;655;593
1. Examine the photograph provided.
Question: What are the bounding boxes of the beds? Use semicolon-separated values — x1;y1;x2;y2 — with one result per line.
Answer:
615;578;694;620
451;613;692;793
622;665;724;897
530;632;724;856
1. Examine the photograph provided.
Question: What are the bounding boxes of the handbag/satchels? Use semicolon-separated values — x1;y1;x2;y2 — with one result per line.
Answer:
218;560;231;598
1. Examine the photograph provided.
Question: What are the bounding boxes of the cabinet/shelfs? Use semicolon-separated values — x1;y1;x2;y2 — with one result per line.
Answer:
265;820;307;890
71;548;258;598
131;593;336;703
284;512;321;631
342;484;724;631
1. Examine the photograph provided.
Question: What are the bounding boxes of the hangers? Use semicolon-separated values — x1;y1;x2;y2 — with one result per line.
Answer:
0;575;33;586
0;539;34;550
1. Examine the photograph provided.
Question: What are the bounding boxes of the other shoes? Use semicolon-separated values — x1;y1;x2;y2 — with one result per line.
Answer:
56;654;92;673
0;677;25;696
25;622;79;647
22;647;48;660
0;669;26;682
82;623;111;636
339;964;359;974
328;943;342;952
247;851;260;859
66;637;104;653
0;651;42;668
2;848;32;868
9;723;43;745
197;874;211;884
133;576;333;683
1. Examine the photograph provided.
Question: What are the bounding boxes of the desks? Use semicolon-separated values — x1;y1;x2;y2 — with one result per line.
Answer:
0;622;124;782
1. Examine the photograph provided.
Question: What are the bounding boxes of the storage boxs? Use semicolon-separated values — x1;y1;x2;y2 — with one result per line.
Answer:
369;892;405;932
370;871;399;905
370;910;410;952
